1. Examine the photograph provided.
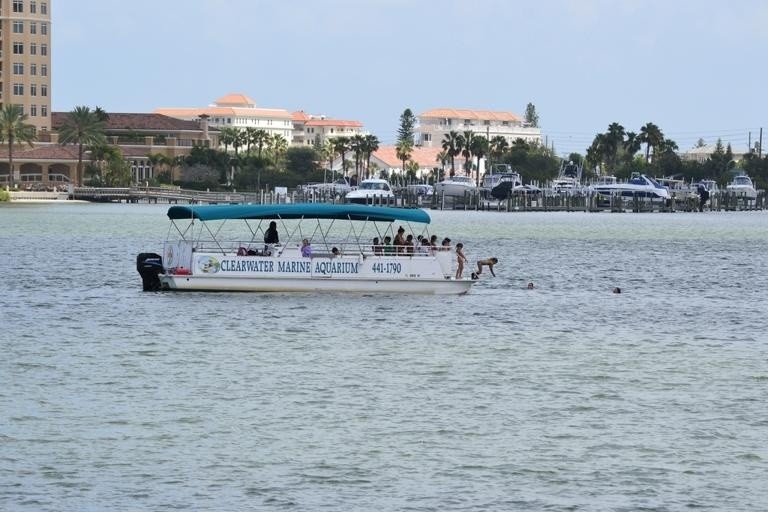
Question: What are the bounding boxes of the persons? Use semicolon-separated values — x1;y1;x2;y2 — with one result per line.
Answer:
472;258;498;279
332;248;341;255
455;243;468;279
372;227;451;255
264;222;279;251
301;239;311;257
613;287;621;293
528;283;534;290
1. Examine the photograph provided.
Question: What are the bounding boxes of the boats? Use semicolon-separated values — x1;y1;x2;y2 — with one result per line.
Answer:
136;203;484;295
